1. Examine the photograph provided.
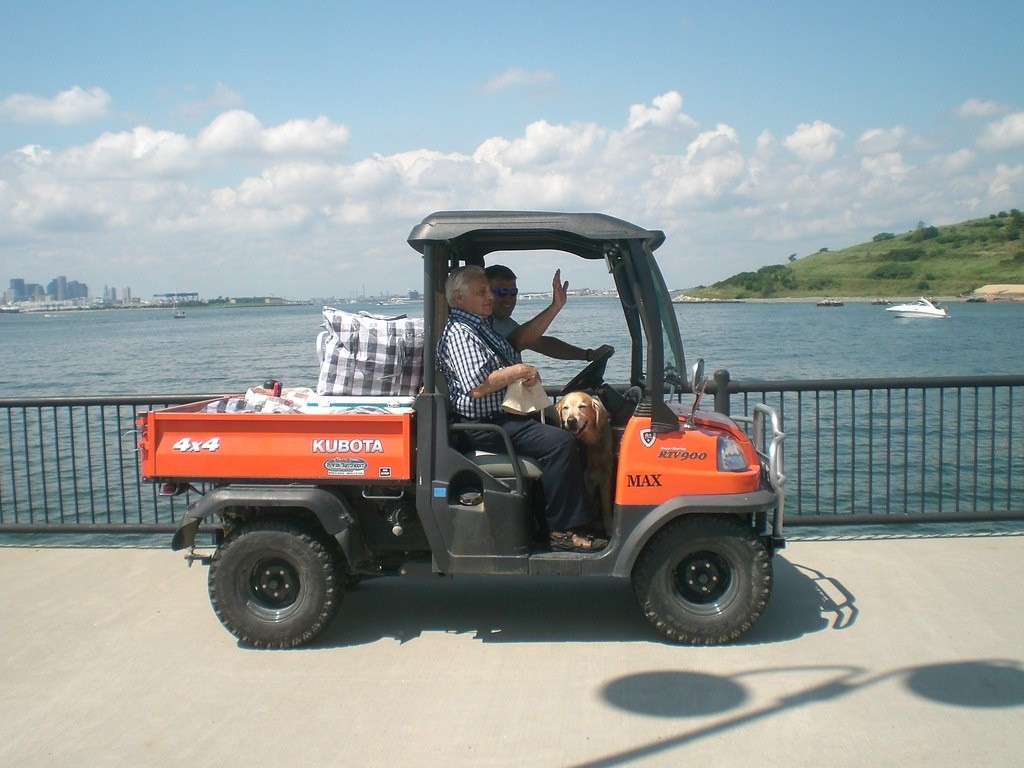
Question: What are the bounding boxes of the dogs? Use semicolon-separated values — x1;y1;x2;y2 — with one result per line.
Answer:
554;391;614;538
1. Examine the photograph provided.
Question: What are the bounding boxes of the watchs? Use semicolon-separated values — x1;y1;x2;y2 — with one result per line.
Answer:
587;349;592;363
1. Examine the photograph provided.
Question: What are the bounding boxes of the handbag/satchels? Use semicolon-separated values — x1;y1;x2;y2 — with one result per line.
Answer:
316;306;425;397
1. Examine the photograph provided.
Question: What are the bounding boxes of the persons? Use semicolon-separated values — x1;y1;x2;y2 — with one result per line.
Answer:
435;265;615;557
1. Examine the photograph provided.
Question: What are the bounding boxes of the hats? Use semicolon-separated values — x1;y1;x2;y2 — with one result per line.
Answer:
502;378;553;416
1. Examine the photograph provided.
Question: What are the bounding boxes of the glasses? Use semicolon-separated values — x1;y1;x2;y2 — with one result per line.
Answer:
490;288;518;296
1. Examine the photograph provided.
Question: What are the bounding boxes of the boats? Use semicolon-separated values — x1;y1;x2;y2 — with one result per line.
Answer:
886;294;951;318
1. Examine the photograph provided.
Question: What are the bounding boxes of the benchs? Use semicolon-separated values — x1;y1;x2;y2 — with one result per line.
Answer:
436;370;542;480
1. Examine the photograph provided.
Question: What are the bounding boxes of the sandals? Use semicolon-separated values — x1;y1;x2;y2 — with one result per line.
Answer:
550;528;609;553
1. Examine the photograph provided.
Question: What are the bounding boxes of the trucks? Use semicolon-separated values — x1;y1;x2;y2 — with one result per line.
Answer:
133;212;789;651
